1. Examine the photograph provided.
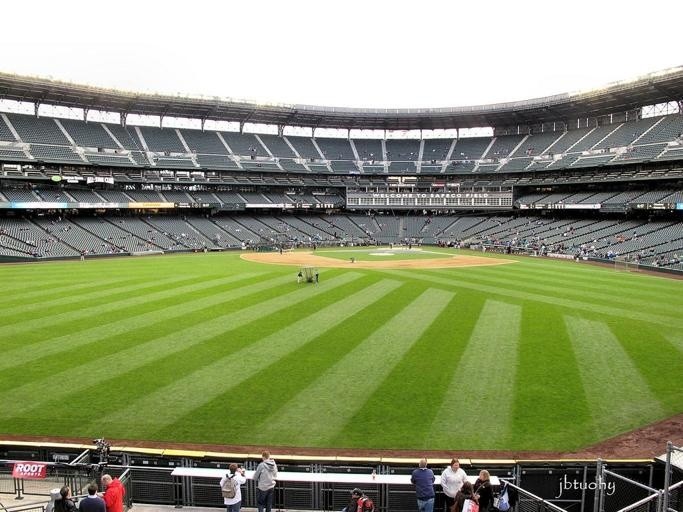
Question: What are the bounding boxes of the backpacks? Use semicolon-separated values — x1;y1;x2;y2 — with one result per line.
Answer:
493;483;510;510
356;497;373;511
221;474;236;499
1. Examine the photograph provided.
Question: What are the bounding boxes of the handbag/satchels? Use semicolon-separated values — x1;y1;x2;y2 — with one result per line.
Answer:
462;499;479;512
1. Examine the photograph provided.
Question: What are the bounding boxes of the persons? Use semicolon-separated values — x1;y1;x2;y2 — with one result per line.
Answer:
297;272;319;282
253;451;278;512
0;215;683;267
54;474;126;512
441;458;494;512
220;464;246;512
343;488;375;512
411;460;435;512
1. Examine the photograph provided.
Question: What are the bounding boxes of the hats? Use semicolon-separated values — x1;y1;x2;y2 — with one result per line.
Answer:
350;488;362;496
